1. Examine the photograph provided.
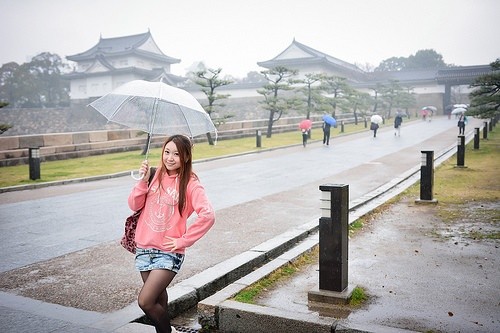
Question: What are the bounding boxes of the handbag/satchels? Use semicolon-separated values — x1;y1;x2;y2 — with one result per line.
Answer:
121;210;142;254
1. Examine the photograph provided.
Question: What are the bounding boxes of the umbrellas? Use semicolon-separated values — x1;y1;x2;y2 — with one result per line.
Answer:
445;105;452;110
87;78;217;181
394;111;407;117
321;115;337;128
451;103;470;114
300;120;312;128
419;105;437;111
371;115;382;124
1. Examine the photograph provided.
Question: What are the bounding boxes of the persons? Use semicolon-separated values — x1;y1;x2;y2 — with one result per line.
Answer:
394;116;402;137
128;134;215;333
370;122;379;137
422;110;433;122
458;114;468;135
301;128;311;148
447;109;452;120
322;122;332;145
